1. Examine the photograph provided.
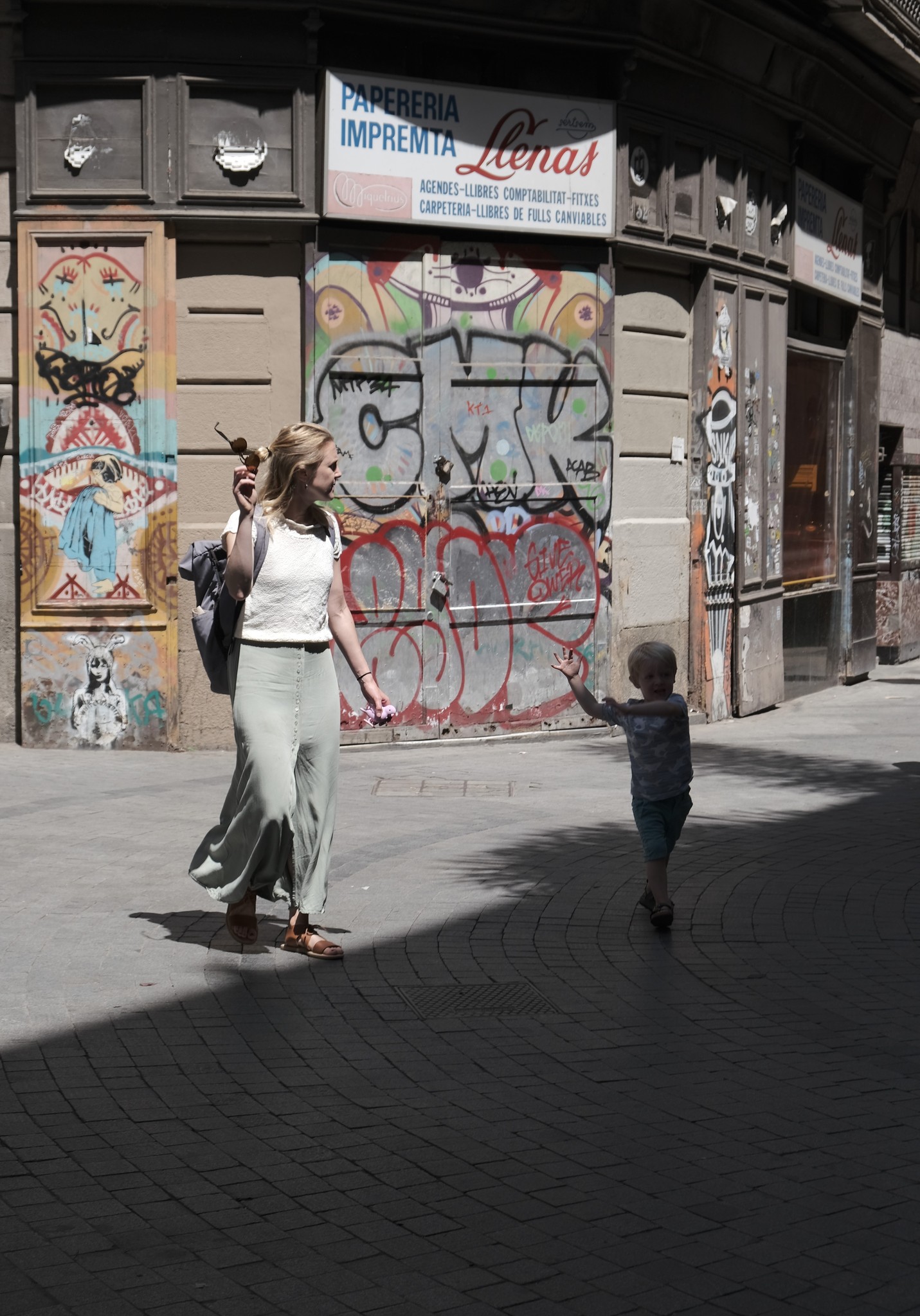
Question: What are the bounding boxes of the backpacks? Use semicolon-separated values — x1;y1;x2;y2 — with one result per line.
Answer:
178;504;335;695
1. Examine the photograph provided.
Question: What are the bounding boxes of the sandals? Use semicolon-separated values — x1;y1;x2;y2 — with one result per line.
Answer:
650;899;674;927
226;894;258;945
280;923;344;959
639;888;655;911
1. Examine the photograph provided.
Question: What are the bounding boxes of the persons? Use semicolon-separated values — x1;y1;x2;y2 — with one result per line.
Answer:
551;641;694;928
188;423;394;961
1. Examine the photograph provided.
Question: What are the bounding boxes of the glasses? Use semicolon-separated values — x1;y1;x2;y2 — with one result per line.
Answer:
214;421;261;472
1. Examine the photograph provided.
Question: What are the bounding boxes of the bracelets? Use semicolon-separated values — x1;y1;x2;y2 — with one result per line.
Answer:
357;671;372;681
